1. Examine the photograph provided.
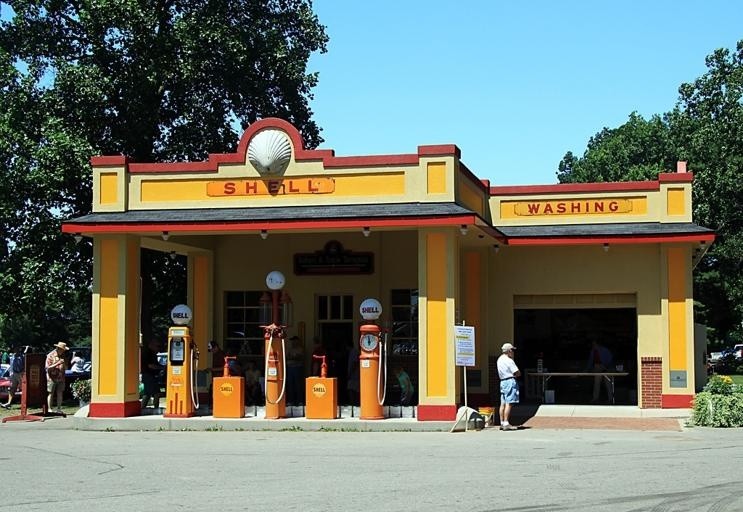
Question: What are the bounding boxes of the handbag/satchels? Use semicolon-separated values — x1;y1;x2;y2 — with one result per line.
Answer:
46;365;65;383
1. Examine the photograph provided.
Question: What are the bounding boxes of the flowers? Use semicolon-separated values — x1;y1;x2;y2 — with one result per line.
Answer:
69;378;90;403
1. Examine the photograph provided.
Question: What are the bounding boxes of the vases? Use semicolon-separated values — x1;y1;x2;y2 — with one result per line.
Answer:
79;399;89;408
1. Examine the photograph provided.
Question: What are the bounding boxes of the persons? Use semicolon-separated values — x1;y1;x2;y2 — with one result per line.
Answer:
141;333;163;409
0;342;85;413
586;340;612;403
496;342;522;430
208;341;224;409
252;335;415;406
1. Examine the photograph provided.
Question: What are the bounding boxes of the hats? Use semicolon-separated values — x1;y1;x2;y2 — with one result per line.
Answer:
501;343;516;350
53;341;69;351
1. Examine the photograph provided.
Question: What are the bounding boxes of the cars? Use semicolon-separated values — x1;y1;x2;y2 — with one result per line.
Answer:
1;364;23;401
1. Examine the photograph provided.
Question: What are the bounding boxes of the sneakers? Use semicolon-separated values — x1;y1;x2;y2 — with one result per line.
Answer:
499;423;517;430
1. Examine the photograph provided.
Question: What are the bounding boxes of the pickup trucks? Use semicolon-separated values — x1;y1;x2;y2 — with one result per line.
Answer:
47;347;91;399
711;344;743;361
